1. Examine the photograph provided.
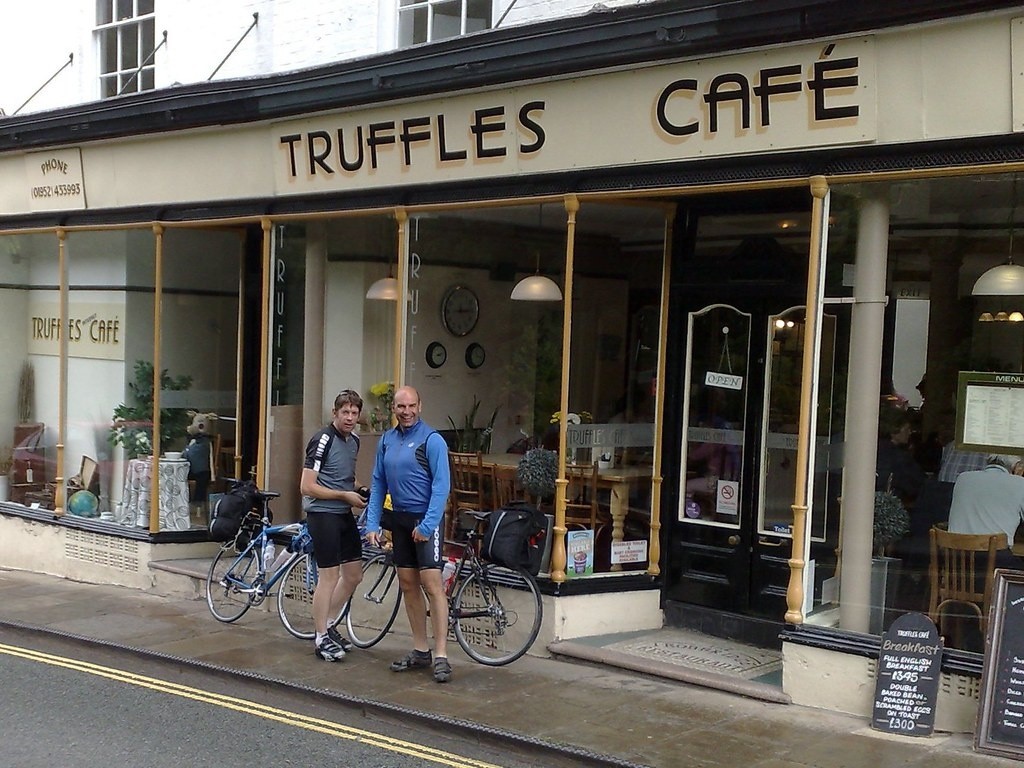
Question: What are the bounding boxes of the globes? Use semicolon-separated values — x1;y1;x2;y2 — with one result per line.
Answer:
68;489;102;519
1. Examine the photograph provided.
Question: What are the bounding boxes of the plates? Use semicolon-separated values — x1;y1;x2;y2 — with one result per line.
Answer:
160;457;187;462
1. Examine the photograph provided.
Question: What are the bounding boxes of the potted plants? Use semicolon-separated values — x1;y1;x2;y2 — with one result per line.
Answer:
869;491;909;637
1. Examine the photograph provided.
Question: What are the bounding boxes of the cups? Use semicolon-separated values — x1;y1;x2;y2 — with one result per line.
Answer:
573;552;588;573
599;461;610;469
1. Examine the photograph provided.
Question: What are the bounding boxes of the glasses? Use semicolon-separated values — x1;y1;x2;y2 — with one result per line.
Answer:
336;390;360;400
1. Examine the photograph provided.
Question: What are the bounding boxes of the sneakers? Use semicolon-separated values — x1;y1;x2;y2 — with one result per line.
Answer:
390;649;432;672
314;637;345;663
314;624;353;652
433;657;452;683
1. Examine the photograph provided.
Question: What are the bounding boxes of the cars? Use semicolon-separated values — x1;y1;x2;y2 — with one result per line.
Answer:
11;422;151;510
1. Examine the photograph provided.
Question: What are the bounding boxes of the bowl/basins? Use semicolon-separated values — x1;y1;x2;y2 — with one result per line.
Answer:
165;452;182;460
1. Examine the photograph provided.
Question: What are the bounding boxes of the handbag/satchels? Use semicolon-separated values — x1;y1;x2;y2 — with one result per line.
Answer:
206;493;272;549
479;500;549;576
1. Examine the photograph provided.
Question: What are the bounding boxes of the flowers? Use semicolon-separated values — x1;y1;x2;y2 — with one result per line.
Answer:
551;411;593;428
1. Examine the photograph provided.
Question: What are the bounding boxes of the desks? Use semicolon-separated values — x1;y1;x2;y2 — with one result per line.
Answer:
451;453;652;571
121;459;191;530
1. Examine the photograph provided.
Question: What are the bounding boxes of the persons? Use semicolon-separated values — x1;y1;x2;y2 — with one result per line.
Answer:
608;388;650;424
300;389;369;661
947;453;1024;593
364;385;451;683
873;406;968;567
687;416;739;503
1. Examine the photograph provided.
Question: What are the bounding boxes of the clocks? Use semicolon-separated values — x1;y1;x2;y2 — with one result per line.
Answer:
440;285;479;338
465;342;486;369
425;342;447;368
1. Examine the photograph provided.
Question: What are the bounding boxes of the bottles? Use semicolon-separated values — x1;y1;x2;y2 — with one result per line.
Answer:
270;547;292;572
442;556;457;594
263;540;275;564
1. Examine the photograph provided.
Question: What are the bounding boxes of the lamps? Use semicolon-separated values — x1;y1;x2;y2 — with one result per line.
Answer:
511;204;563;302
366;266;412;301
972;213;1024;322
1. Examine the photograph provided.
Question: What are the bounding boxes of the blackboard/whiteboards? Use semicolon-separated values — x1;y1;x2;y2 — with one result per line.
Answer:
975;569;1024;762
872;612;945;737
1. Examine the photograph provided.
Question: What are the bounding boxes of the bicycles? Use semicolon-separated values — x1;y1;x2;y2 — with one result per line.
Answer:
346;508;543;667
204;491;353;640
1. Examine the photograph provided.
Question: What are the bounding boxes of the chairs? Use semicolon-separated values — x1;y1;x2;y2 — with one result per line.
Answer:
185;434;222;525
445;448;493;559
554;461;630;571
489;463;544;514
927;525;1008;655
